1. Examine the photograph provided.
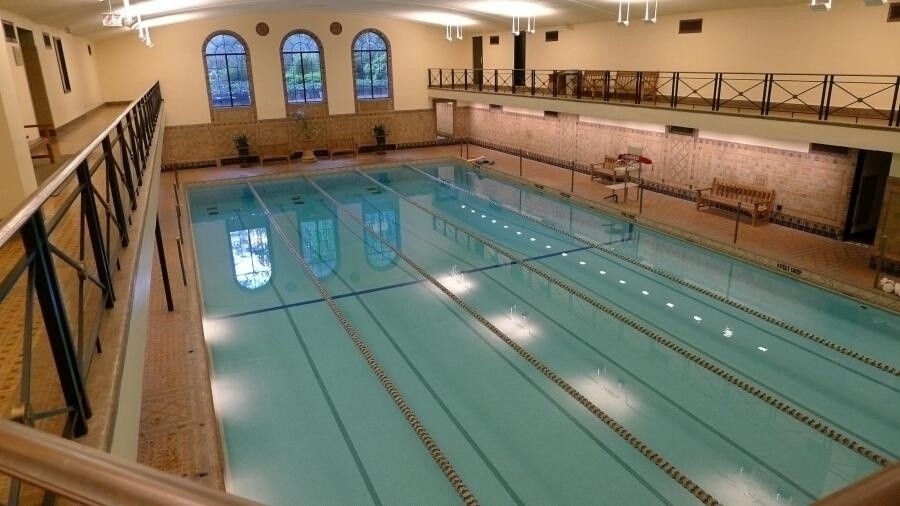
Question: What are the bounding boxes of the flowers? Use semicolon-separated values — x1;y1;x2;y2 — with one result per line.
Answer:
292;106;318;163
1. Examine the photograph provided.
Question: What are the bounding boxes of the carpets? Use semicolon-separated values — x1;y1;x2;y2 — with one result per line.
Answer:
30;152;80;196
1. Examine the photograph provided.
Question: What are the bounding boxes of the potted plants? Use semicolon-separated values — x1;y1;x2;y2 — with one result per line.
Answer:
373;122;389;155
232;133;252;168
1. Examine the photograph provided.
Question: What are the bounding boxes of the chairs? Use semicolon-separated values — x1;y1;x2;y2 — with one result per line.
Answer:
604;144;643;203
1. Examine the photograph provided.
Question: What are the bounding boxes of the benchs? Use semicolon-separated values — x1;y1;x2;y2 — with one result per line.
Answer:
571;69;611;98
325;136;358;160
696;177;775;227
591;155;631;185
256;142;291;167
613;71;659;107
23;123;56;165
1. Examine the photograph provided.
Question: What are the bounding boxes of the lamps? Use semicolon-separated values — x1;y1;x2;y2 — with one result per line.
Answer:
100;0;154;49
616;0;658;26
509;15;536;37
444;25;463;42
